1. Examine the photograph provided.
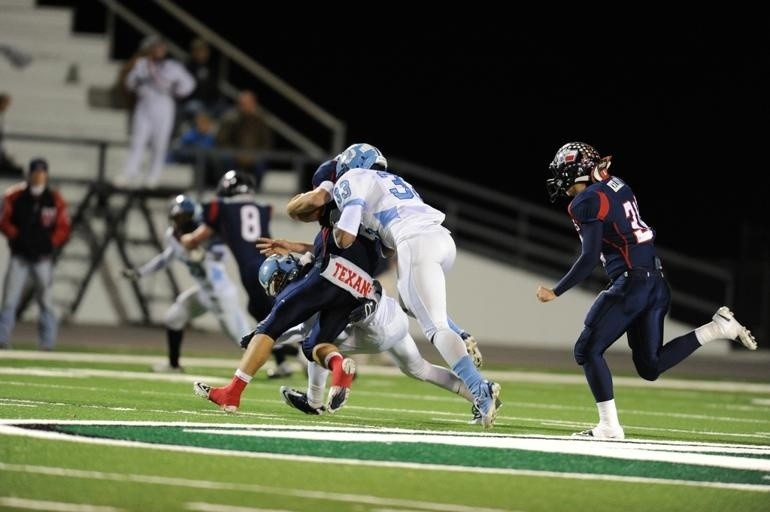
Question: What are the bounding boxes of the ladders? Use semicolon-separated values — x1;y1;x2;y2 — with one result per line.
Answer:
15;182;192;329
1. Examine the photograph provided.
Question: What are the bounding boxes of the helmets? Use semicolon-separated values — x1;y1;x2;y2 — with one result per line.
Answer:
336;143;388;179
258;253;299;297
214;169;254;197
168;194;195;219
547;141;601;203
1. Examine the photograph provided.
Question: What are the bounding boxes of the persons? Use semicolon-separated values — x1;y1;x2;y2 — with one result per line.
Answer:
535;141;757;442
0;160;71;352
121;140;503;427
124;35;268;190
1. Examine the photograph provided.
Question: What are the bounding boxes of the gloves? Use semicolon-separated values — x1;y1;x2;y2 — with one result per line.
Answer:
348;297;377;322
240;330;256;349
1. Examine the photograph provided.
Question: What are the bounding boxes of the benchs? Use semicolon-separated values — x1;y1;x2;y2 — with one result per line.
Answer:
0;0;321;196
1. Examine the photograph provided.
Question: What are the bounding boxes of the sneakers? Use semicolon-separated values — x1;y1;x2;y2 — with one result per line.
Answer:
471;380;503;428
280;385;326;415
193;382;238;414
572;425;625;440
267;361;293;378
326;358;356;413
152;364;184;374
712;306;758;351
460;332;482;368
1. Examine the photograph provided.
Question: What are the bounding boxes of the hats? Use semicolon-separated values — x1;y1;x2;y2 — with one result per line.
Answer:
29;159;47;172
